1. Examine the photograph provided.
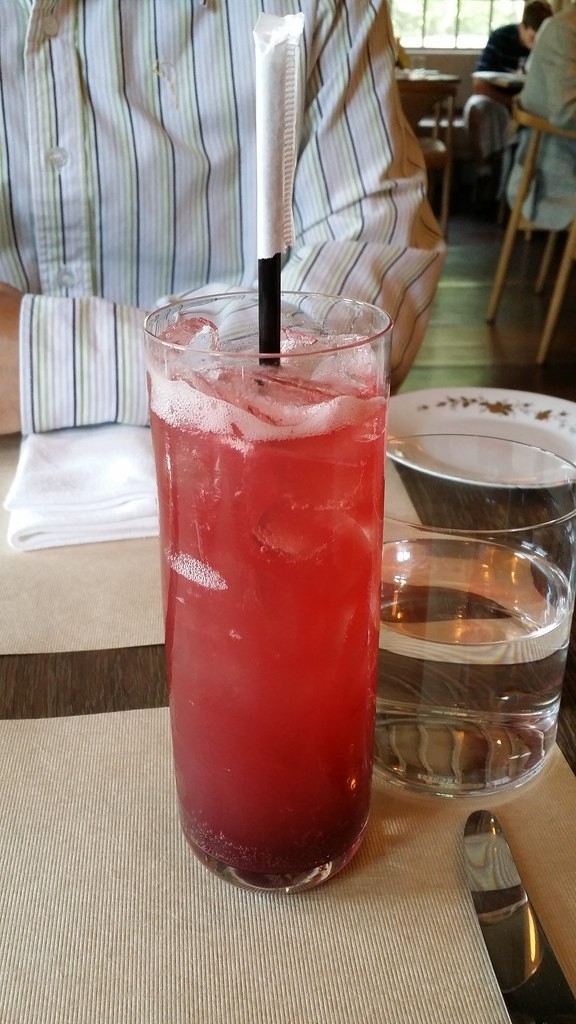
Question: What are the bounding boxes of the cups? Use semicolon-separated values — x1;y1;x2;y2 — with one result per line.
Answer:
144;290;393;893
384;431;576;798
408;56;426;81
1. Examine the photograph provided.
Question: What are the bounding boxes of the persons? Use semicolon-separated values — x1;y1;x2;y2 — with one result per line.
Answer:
460;0;554;209
506;0;576;234
0;0;448;442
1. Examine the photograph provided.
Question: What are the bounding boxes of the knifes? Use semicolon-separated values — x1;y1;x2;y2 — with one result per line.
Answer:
464;809;576;1024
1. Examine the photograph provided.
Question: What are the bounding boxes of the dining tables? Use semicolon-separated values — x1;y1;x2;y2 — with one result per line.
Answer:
0;412;576;1023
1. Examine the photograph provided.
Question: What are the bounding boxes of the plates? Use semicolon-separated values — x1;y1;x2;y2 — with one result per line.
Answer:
386;387;576;541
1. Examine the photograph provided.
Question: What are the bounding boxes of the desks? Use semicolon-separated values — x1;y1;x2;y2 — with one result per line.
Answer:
471;72;522;104
396;68;463;131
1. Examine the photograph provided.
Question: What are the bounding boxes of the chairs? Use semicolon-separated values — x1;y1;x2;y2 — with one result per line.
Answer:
415;93;456;243
485;95;576;367
